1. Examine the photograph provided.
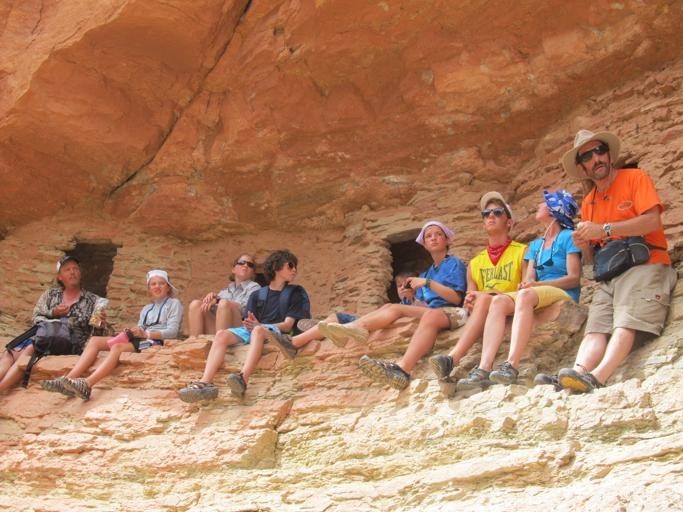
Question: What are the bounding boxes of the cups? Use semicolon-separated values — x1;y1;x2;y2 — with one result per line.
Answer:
88;298;109;327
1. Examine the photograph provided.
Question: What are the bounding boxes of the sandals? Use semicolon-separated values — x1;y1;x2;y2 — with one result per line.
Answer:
429;355;519;389
226;372;247;401
359;355;410;388
179;380;218;403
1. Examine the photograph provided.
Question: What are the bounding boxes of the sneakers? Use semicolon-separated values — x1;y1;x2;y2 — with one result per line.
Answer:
38;376;91;401
318;321;370;348
298;319;318;330
265;327;297;358
534;368;606;394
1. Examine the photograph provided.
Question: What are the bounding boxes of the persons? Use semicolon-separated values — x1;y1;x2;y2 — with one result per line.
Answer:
177;250;310;403
262;270;418;360
0;256;115;392
317;221;466;348
455;188;582;388
358;191;529;391
38;269;184;400
189;254;262;338
533;129;678;392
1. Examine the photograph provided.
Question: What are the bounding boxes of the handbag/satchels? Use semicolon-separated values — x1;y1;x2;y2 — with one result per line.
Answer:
34;315;81;356
592;236;650;282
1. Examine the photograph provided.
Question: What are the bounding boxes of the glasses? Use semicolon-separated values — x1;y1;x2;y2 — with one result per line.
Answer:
481;207;508;221
576;144;609;163
532;240;555;271
284;261;297;270
236;260;255;268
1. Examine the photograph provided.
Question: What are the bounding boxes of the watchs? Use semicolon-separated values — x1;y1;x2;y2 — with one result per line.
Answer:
603;223;610;236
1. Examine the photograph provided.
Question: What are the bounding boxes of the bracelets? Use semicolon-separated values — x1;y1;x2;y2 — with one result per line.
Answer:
426;278;431;288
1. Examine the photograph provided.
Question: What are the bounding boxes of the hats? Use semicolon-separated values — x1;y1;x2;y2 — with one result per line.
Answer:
415;221;456;246
561;129;622;180
480;191;515;233
56;255;81;273
146;269;178;296
543;189;579;230
